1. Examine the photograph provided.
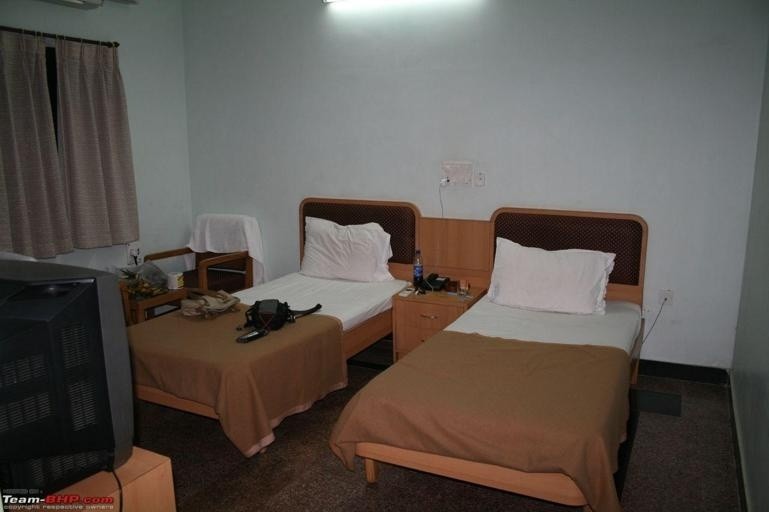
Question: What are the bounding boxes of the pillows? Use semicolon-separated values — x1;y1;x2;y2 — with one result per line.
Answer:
487;234;617;315
298;214;392;283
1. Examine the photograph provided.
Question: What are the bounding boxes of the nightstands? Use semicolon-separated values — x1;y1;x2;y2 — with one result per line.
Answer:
391;282;487;364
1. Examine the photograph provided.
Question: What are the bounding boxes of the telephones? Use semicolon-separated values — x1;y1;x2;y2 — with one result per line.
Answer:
424;273;450;291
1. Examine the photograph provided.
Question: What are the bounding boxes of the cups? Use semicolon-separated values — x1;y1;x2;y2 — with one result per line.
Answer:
457;280;469;298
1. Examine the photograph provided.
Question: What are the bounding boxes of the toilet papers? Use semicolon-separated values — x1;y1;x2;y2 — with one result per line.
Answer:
167;271;184;289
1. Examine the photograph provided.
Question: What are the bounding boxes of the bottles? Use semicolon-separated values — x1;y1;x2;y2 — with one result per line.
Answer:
413;250;424;287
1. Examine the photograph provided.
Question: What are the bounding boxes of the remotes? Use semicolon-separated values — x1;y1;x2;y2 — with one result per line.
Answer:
399;287;416;298
236;328;267;343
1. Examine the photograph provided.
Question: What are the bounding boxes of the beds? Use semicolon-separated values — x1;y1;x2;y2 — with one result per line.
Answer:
344;209;648;511
132;198;419;449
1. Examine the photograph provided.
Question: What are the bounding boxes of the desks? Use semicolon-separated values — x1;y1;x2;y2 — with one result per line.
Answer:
122;280;189;325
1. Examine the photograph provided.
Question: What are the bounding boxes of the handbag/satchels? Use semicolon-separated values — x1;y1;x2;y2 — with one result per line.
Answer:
244;299;289;330
180;288;240;320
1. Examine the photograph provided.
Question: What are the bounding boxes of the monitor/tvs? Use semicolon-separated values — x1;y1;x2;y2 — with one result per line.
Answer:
0;259;136;507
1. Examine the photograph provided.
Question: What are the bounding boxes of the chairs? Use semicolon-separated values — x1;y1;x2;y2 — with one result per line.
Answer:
143;214;257;308
